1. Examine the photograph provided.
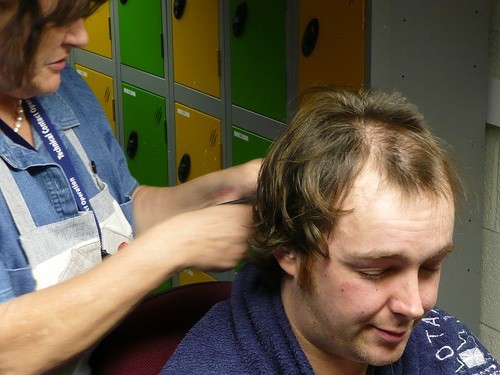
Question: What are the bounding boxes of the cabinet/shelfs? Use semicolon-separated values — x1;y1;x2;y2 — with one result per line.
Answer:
68;0;373;296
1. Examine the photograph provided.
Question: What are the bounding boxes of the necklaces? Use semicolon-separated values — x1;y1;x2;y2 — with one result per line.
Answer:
13;99;24;133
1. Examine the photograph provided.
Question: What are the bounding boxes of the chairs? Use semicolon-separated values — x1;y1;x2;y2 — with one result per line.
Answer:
74;282;233;375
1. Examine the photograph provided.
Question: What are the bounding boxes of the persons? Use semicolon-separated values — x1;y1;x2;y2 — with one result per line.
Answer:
157;82;500;375
0;0;267;375
